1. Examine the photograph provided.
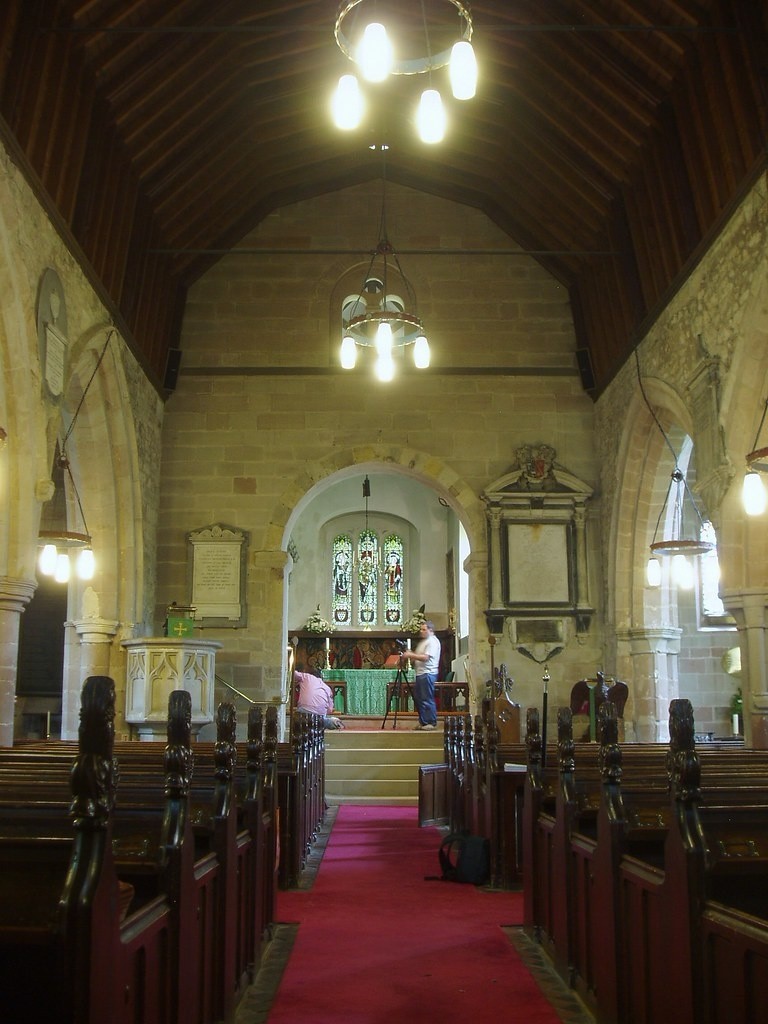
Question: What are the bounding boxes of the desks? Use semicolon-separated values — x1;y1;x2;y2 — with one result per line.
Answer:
444;700;768;1024
386;682;469;712
0;676;327;1024
324;681;347;715
319;669;414;716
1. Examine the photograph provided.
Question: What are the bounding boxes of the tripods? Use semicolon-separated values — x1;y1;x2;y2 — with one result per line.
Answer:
382;651;418;730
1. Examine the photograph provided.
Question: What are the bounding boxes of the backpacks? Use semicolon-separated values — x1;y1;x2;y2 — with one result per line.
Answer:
439;833;492;887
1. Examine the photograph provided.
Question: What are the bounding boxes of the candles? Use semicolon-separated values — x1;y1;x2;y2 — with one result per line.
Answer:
326;637;330;650
407;639;411;650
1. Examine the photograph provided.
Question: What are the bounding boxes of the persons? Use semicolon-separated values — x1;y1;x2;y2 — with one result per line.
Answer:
403;621;441;731
295;668;346;730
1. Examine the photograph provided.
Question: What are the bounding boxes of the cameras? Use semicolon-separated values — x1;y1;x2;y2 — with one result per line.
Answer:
395;639;407;652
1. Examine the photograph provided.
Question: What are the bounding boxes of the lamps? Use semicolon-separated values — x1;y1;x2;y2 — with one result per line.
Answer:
741;398;768;516
338;149;431;384
39;456;97;584
646;467;720;587
328;0;479;145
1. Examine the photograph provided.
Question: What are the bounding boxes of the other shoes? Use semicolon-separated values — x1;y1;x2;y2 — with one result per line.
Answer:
415;725;423;730
421;724;436;730
